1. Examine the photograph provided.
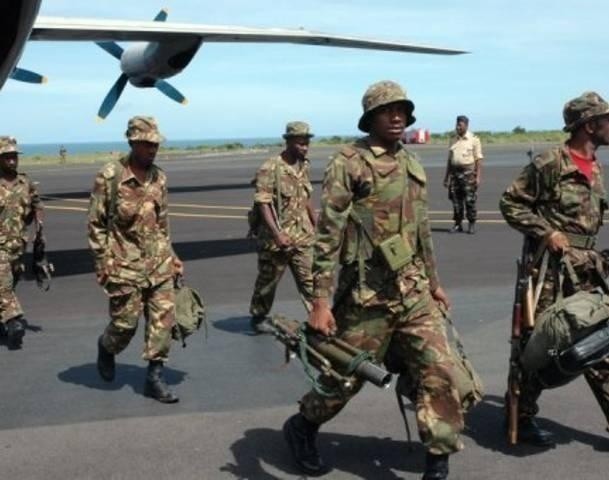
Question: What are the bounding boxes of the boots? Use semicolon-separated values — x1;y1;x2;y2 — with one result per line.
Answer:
422;453;448;480
283;412;325;475
5;317;25;338
145;361;178;403
250;316;275;333
451;221;463;232
98;334;115;381
469;220;476;234
505;391;555;445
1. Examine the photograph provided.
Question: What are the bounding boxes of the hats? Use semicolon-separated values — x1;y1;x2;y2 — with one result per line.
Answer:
457;116;469;123
126;116;163;144
283;122;314;139
563;92;609;132
0;137;22;154
358;81;415;132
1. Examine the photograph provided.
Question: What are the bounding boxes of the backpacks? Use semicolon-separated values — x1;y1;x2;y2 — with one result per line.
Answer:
519;248;609;390
173;276;208;348
247;200;263;238
396;301;485;453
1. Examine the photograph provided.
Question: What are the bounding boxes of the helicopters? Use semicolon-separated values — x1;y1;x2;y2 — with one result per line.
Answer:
1;0;471;119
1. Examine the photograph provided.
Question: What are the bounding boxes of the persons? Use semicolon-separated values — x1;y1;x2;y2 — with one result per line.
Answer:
1;136;51;350
500;90;609;449
81;118;185;404
281;81;478;480
442;115;483;233
248;121;322;334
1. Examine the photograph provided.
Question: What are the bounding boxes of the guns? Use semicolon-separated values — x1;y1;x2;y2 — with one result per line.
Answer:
505;258;531;443
32;224;54;287
